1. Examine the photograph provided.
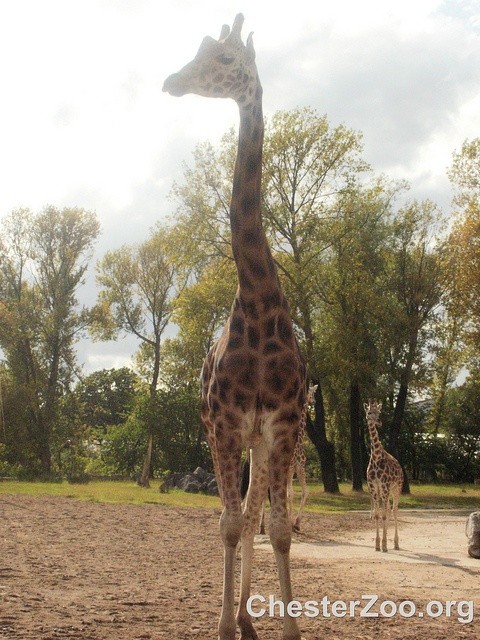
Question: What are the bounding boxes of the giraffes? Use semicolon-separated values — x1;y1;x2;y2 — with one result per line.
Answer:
160;11;308;640
363;398;405;554
